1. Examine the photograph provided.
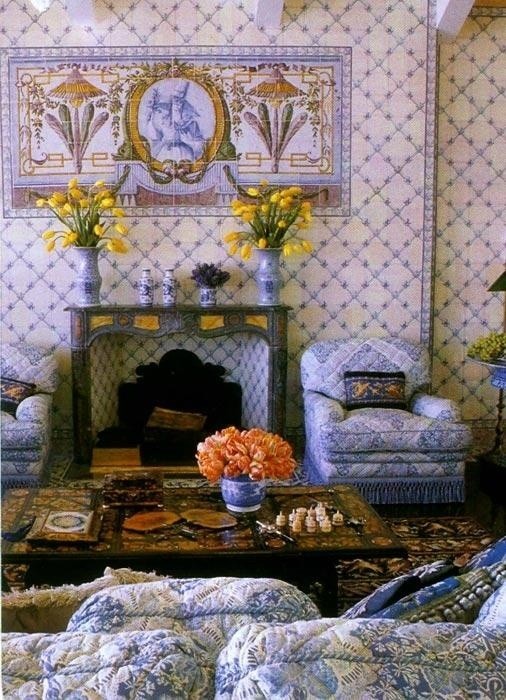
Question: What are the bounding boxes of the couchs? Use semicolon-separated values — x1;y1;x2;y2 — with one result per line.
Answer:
1;567;504;698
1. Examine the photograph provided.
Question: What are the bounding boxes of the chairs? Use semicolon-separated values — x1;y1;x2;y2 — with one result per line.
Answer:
0;341;59;488
300;335;474;519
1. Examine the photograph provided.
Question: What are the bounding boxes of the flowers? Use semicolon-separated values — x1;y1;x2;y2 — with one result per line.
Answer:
468;331;505;361
189;263;230;287
192;425;298;485
224;180;315;260
29;163;134;255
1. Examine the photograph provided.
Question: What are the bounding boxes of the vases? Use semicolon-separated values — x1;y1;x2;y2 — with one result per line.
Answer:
198;286;218;306
468;355;505;389
139;266;176;307
220;475;267;513
72;245;103;307
254;247;284;306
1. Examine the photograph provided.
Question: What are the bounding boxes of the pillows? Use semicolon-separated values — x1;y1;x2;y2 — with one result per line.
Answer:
67;576;322;631
0;569;171;634
0;375;37;416
342;371;408;411
340;558;506;625
